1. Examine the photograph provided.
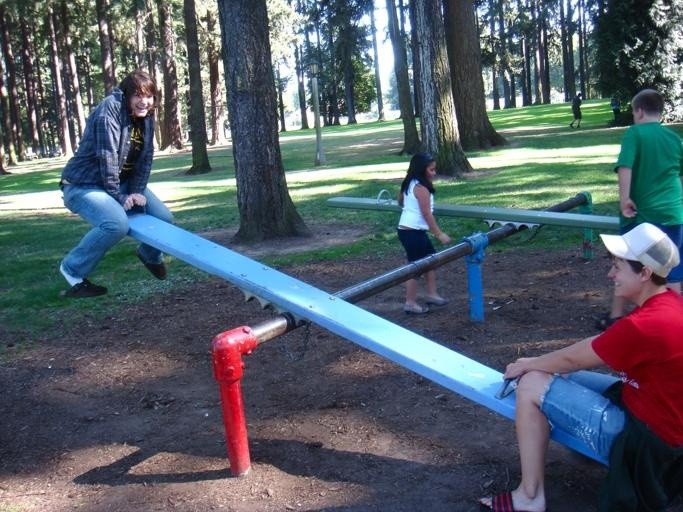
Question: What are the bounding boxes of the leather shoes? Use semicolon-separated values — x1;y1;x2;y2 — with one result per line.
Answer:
423;294;448;307
403;304;428;315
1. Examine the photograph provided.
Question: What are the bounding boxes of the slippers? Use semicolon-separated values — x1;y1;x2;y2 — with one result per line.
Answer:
479;491;547;512
135;249;166;280
57;280;107;298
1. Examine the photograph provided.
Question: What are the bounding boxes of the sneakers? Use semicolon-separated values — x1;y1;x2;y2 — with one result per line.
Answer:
594;311;620;331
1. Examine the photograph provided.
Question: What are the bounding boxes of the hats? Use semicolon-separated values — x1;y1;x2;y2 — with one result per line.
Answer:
598;221;680;279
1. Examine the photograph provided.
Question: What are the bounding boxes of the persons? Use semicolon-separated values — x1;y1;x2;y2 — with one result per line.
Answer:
56;69;174;299
592;88;682;331
568;92;582;130
396;150;452;316
608;93;622;118
474;221;682;512
